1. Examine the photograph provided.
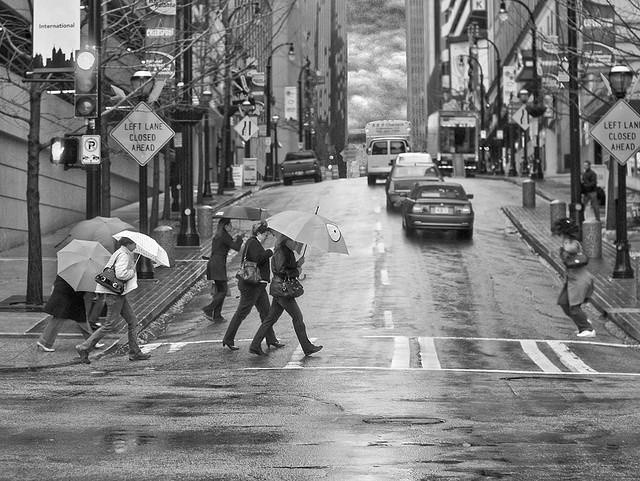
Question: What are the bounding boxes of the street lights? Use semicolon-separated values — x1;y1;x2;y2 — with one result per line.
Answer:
201;86;213;197
176;81;185;208
468;35;506;176
604;58;635;279
518;87;531;176
458;53;486;172
500;0;545;178
266;41;296;183
129;68;158;278
272;113;281;180
241;98;251;184
303;121;311;149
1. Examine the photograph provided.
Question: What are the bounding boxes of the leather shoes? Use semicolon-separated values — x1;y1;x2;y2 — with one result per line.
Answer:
249;349;267;357
304;345;322;356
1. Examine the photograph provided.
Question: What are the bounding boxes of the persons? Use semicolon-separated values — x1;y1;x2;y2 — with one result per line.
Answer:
75;236;151;364
248;234;324;356
223;225;286;350
550;218;597;338
36;275;105;352
84;292;106;330
202;219;245;323
579;160;600;220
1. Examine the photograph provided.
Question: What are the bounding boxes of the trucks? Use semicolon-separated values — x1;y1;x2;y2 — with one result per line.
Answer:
426;108;480;178
363;118;415;152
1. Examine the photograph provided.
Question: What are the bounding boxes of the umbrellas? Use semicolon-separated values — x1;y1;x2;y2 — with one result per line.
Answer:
56;239;112;294
265;205;350;281
212;205;269;236
69;216;137;254
111;230;171;273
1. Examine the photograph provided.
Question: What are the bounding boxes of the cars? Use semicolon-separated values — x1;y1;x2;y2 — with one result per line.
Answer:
392;151;433;165
384;160;445;209
398;181;475;241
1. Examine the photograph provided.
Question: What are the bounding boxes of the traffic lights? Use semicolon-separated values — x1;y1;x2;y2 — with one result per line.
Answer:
49;136;78;165
75;45;102;119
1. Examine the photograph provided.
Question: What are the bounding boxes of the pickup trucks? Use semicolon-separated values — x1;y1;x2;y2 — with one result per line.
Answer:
280;148;322;185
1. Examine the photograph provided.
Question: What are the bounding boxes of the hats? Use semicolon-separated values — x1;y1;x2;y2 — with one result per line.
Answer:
551;218;578;234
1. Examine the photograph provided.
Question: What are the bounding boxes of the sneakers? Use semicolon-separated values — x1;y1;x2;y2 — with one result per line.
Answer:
575;329;598;338
76;345;91;364
129;353;151;361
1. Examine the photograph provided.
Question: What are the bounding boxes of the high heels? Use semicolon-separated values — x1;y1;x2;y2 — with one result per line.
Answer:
95;342;104;348
35;340;56;352
267;341;285;350
222;341;239;350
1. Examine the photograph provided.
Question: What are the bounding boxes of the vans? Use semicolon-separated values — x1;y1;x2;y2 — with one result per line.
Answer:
366;137;412;185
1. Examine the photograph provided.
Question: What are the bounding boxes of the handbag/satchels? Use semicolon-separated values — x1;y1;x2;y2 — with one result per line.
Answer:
561;252;589;266
236;257;261;283
269;276;303;298
95;265;126;294
